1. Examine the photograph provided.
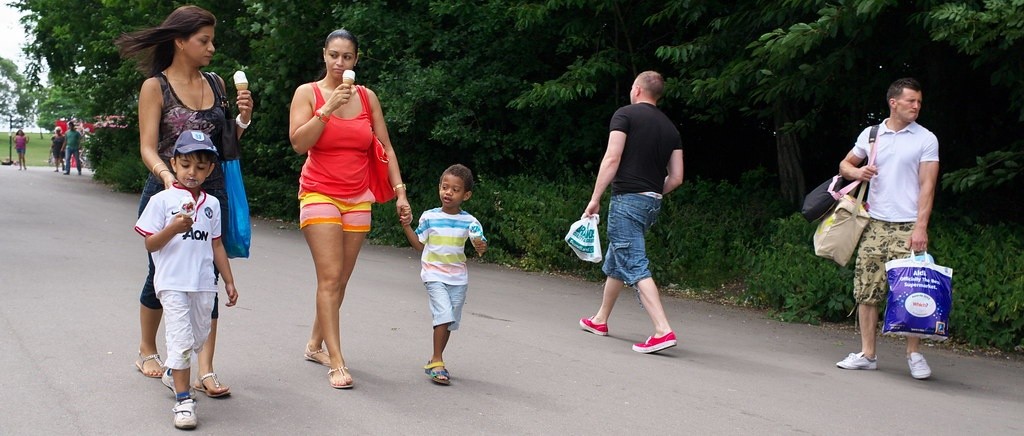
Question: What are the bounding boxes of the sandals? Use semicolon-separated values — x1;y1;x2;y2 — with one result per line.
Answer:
328;366;353;388
193;372;231;397
424;361;449;384
425;360;448;374
135;350;166;377
304;343;331;367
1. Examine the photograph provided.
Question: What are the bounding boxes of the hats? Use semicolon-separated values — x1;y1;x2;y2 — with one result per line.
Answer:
173;129;220;157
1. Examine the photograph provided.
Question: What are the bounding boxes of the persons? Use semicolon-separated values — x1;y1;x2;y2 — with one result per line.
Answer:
289;28;413;389
578;72;683;352
50;127;65;172
61;121;82;175
13;130;29;170
836;78;940;379
135;131;238;429
400;164;487;384
115;6;253;397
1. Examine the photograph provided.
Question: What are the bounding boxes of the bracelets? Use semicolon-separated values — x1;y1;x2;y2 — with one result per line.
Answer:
393;184;406;193
315;109;331;124
153;163;168;178
236;114;251;129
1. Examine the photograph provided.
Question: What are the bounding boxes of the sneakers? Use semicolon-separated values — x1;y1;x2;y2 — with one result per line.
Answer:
173;395;198;429
908;352;932;378
579;316;608;336
161;368;196;399
632;331;677;353
836;352;878;370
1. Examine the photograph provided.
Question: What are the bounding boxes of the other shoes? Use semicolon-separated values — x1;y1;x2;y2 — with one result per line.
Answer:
78;170;81;175
54;170;58;172
63;171;70;175
62;165;65;171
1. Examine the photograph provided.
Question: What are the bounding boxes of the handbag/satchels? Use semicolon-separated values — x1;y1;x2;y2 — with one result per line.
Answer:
565;213;603;262
223;160;251;259
369;133;397;202
802;172;853;222
882;249;953;342
211;72;240;161
813;194;871;266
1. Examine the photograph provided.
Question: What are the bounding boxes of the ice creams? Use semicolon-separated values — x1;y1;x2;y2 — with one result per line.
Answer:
342;70;355;90
180;200;196;219
233;70;249;91
469;221;482;257
